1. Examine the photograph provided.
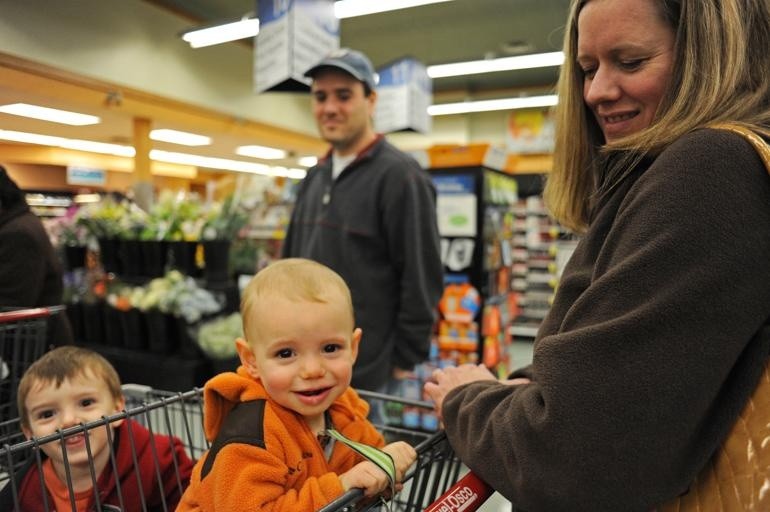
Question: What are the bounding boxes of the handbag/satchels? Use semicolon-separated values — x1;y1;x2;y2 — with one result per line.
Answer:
36;270;74;353
658;364;770;512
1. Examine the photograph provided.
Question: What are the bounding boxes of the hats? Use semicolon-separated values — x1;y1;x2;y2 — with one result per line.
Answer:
304;48;376;93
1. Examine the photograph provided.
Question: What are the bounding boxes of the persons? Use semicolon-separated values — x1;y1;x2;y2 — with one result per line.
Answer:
173;255;417;512
278;47;445;434
423;1;770;511
0;345;199;512
1;163;78;475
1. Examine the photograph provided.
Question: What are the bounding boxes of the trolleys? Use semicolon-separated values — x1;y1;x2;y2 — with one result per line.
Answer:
1;382;495;511
0;301;68;489
